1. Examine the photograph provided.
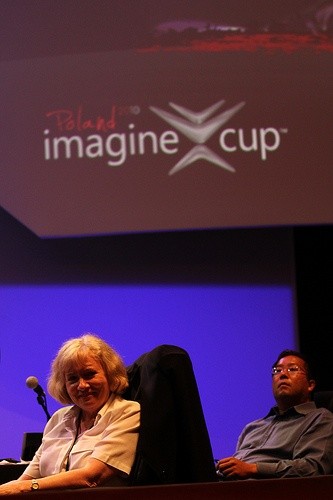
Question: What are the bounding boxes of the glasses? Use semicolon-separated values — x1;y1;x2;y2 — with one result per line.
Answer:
271;365;301;375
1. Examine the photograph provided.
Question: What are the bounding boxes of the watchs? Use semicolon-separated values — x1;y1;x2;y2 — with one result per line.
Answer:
31;478;39;490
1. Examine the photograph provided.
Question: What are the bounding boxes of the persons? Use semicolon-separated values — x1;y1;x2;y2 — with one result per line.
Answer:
216;349;333;479
0;332;142;496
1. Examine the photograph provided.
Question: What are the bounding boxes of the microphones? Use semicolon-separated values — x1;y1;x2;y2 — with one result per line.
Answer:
25;376;45;396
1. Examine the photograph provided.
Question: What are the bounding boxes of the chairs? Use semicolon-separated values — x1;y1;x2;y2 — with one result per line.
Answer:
121;344;218;487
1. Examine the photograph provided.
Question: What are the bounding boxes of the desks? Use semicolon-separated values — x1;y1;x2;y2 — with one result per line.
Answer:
0;459;29;485
